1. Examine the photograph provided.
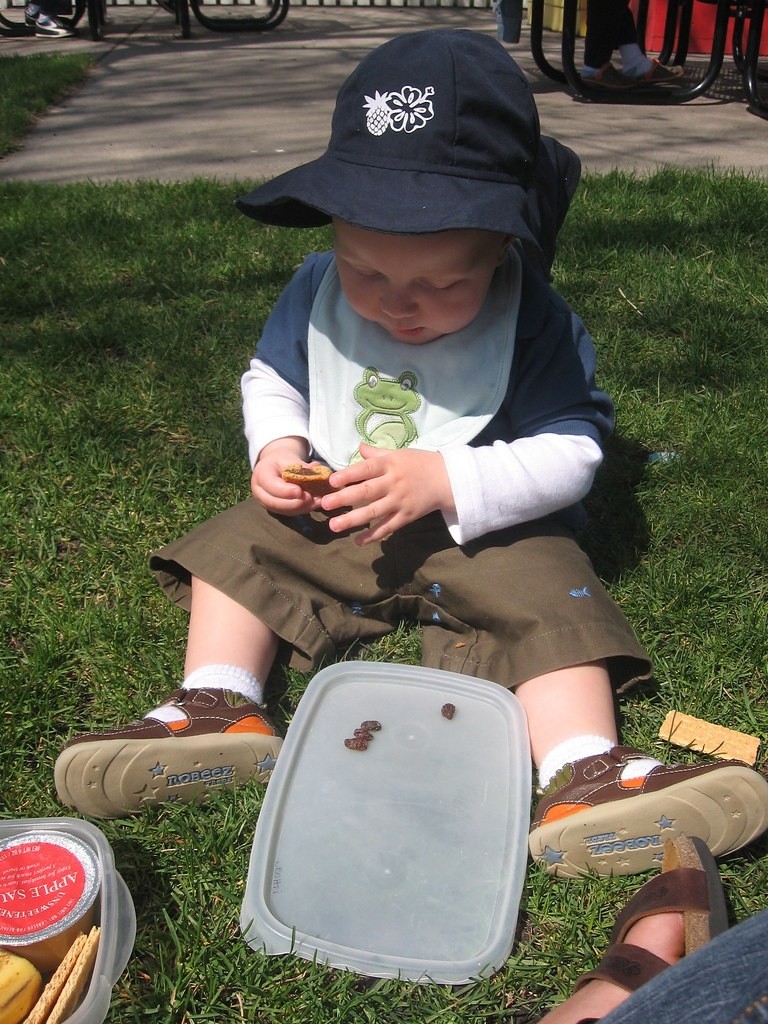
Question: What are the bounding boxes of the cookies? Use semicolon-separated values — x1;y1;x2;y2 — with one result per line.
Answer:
20;925;102;1024
281;465;350;497
658;710;761;767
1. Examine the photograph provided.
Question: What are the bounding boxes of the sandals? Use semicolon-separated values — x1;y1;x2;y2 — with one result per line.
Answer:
54;689;285;819
573;837;730;993
527;746;767;881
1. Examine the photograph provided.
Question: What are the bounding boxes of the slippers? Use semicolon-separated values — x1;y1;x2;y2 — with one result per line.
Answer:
576;64;637;88
637;59;683;83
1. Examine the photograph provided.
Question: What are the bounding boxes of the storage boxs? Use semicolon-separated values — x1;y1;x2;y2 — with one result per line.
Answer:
0;816;119;1024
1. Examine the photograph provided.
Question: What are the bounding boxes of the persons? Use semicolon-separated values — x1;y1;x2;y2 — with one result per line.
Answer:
580;0;685;90
54;28;768;880
535;834;768;1024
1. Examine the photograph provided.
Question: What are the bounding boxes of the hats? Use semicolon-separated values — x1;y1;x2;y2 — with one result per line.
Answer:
235;28;582;284
